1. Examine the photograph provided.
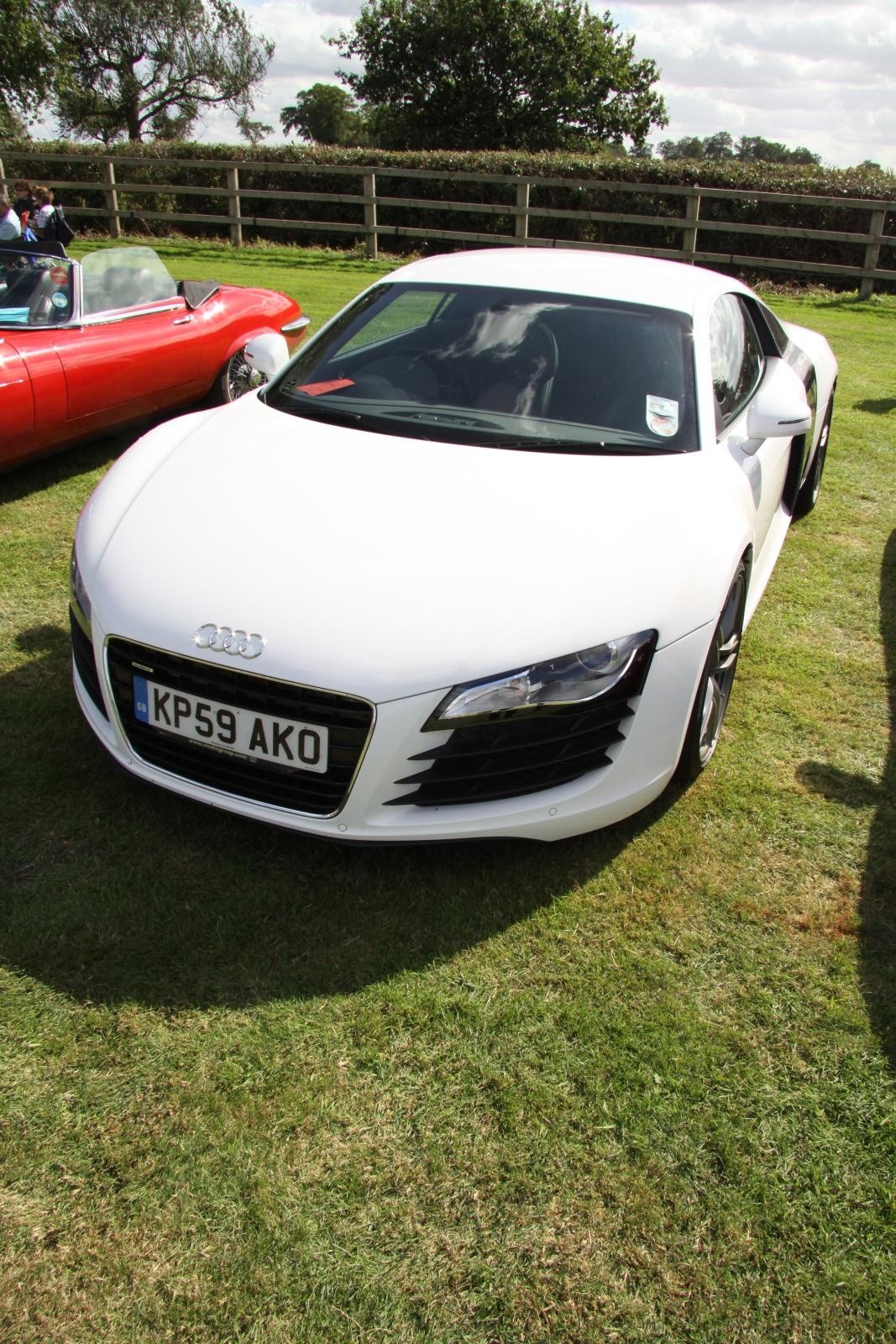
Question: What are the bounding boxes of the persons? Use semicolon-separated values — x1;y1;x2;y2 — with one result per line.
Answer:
0;193;21;242
12;177;36;226
27;185;56;239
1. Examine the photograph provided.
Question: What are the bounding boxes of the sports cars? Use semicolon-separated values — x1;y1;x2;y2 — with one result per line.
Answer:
65;246;844;855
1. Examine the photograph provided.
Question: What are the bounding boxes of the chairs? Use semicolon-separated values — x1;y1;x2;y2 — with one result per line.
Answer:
476;319;560;417
4;267;60;322
102;265;155;310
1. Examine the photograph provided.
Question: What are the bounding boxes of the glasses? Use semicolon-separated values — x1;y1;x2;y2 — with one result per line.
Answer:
16;188;25;192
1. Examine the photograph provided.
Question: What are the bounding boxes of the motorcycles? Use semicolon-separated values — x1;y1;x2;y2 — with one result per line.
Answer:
0;236;310;473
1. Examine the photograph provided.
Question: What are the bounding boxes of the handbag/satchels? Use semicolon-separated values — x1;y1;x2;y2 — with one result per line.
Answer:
54;206;76;247
